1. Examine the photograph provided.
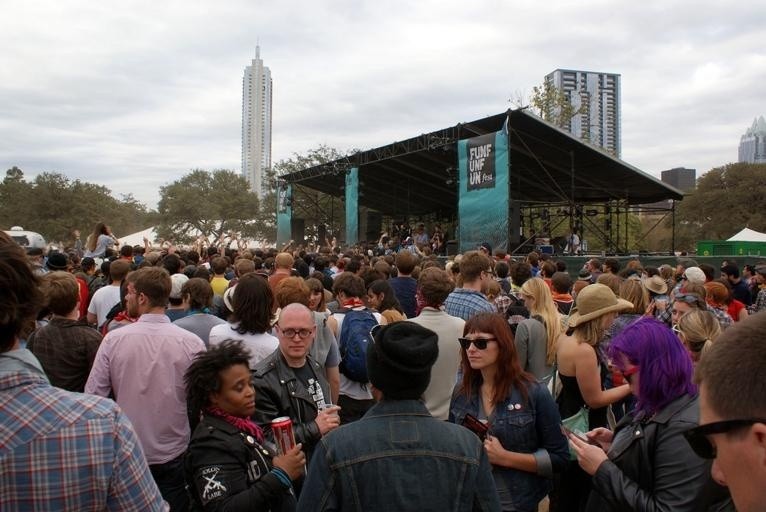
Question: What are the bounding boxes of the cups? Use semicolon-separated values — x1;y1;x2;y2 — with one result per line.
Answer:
321;403;339;422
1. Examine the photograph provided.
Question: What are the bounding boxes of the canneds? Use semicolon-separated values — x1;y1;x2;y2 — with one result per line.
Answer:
270;416;296;457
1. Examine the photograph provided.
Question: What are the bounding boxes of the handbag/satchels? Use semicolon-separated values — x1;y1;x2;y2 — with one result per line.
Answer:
559;406;590;460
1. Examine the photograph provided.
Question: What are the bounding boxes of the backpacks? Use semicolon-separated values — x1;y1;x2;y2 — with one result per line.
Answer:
334;307;380;385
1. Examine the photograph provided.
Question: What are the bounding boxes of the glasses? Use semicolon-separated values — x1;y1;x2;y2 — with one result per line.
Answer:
683;417;766;459
279;325;311;339
458;336;498;351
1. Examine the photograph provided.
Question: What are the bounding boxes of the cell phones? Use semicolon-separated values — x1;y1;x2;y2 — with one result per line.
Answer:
573;427;602;448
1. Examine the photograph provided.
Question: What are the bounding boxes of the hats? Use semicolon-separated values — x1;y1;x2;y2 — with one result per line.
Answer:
685;266;706;285
568;282;635;327
168;273;190;298
643;275;668;294
369;321;439;383
754;264;766;275
223;283;239;314
46;252;67;270
